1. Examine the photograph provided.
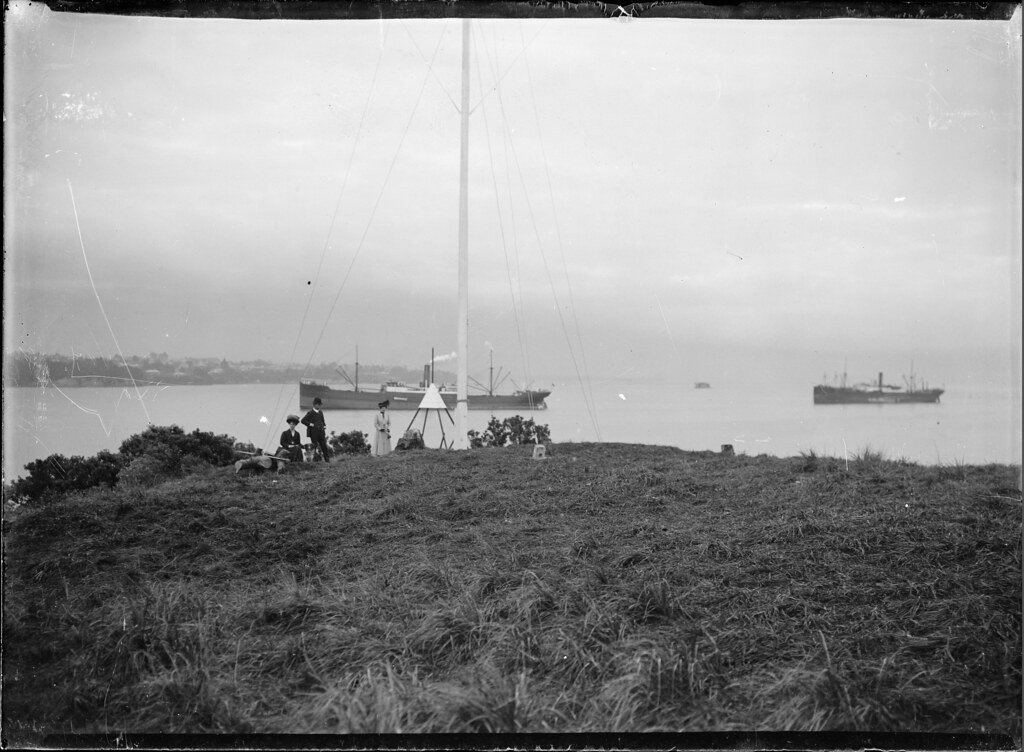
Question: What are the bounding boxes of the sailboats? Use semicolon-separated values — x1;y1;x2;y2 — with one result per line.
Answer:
294;11;552;413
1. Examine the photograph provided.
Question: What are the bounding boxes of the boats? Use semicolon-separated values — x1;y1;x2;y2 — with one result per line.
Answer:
812;363;944;405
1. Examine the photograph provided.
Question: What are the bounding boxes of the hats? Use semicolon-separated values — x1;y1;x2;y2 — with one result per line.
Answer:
312;397;324;405
287;415;299;423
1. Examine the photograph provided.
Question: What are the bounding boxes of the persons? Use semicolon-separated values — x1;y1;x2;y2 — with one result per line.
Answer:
371;400;391;456
301;397;330;462
280;414;303;463
